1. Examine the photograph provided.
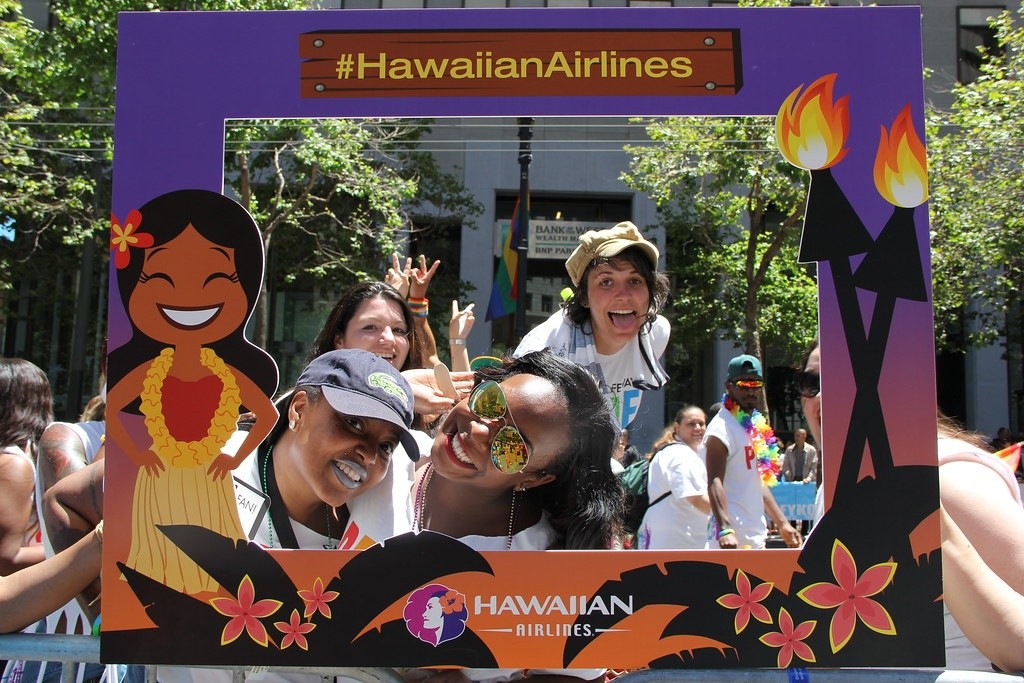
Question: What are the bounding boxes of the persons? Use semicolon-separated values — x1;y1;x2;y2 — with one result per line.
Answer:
0;342;107;683
699;355;802;549
610;428;646;470
313;352;625;683
40;347;419;683
707;402;823;538
634;354;713;550
508;220;670;437
307;252;476;439
797;337;1024;675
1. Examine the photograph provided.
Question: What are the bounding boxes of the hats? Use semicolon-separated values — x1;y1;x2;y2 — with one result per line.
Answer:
725;354;763;380
295;348;421;463
565;220;660;288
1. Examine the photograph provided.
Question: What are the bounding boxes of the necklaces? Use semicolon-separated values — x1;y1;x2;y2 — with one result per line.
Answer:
263;441;333;550
722;394;781;488
412;458;522;549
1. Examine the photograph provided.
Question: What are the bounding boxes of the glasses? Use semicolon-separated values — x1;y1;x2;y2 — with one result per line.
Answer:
732;381;765;393
792;371;820;398
467;379;530;477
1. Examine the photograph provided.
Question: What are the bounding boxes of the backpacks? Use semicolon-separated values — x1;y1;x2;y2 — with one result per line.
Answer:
609;438;689;532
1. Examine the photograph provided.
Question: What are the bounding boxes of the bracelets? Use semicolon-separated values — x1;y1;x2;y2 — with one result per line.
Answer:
717;528;736;538
448;338;466;345
406;297;430;318
95;518;104;541
